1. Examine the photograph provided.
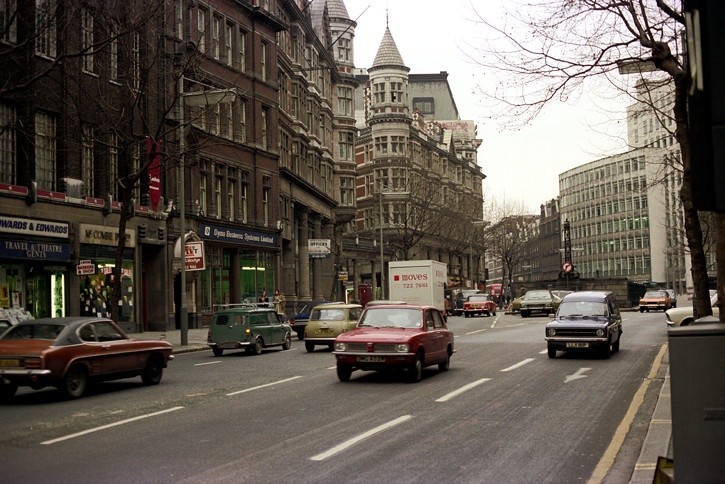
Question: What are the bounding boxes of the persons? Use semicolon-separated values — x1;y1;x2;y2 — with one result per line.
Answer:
506;294;510;305
56;309;62;317
498;293;505;305
273;289;285;324
258;291;269;308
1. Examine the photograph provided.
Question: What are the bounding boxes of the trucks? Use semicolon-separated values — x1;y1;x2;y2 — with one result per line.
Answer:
485;284;504;300
389;260;454;322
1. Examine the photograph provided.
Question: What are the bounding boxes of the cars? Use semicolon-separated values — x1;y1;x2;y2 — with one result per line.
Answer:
639;290;676;312
664;293;720;327
206;302;291;355
453;290;497;318
512;290;572;318
292;300;456;384
689;290;719;307
0;317;175;401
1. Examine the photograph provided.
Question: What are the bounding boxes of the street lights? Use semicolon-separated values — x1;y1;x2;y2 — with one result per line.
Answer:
378;190;411;301
470;220;491;290
177;74;235;344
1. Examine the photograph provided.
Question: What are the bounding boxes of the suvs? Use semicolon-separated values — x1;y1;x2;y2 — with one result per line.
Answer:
545;291;623;359
